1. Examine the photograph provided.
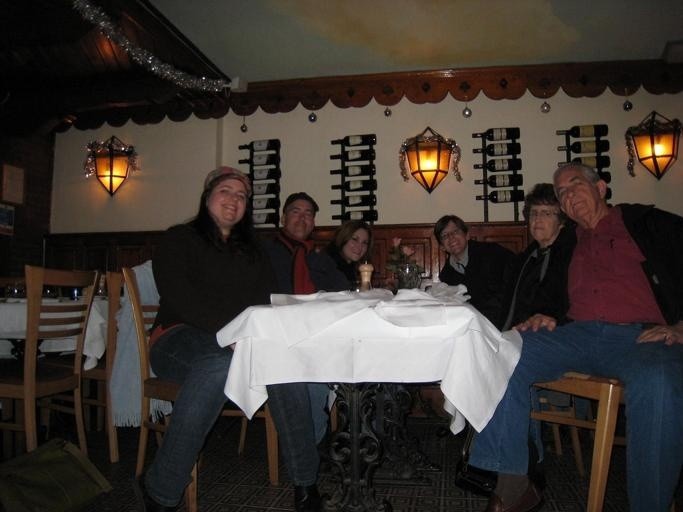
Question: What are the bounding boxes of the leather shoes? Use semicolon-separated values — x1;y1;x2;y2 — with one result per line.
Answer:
484;476;545;512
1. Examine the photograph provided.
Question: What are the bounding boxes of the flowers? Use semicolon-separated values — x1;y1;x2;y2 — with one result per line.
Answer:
384;237;425;273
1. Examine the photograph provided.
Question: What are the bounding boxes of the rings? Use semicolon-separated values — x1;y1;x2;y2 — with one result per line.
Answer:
664;331;667;342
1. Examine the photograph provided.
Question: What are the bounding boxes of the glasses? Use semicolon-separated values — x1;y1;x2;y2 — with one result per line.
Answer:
439;227;460;241
527;209;560;220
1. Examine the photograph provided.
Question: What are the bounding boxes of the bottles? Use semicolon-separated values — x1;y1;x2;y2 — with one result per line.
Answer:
555;124;612;201
328;133;380;222
470;127;525;203
358;264;374;293
236;139;280;227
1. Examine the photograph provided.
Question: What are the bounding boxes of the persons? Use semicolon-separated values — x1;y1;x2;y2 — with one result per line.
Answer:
314;218;372;293
132;165;323;511
455;182;591;497
470;164;683;512
435;215;520;332
254;191;350;485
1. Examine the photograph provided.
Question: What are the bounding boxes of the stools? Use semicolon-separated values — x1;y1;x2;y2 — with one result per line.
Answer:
528;369;676;512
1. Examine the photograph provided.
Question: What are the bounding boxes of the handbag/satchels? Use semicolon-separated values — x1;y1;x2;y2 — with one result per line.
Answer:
454;458;547;497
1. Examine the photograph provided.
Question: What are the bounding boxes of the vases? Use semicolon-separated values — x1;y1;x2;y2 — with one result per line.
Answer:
398;265;420;289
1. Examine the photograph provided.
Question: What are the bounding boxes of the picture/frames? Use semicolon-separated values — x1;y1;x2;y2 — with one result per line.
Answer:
0;201;18;241
0;161;27;208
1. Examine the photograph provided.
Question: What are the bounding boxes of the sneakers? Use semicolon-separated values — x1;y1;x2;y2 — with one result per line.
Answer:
134;474;177;511
293;483;323;512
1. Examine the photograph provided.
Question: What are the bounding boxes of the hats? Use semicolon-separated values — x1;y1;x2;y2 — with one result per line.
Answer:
204;167;253;198
283;192;319;213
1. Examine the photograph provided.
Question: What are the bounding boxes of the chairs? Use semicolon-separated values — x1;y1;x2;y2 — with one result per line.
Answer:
0;264;100;459
82;274;107;297
121;267;279;512
238;384;338;458
40;269;126;463
0;275;26;298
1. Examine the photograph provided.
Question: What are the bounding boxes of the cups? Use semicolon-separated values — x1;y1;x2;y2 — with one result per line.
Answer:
1;277;108;299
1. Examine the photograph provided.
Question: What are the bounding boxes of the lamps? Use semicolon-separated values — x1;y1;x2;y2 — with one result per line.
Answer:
632;111;679;180
405;127;452;193
93;136;130;196
